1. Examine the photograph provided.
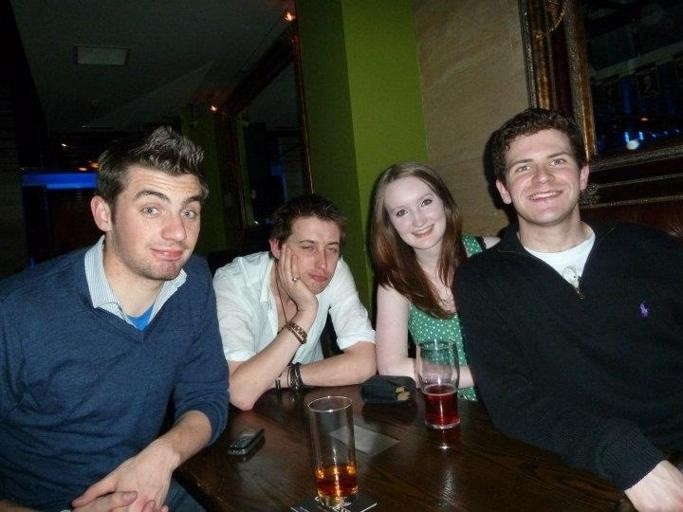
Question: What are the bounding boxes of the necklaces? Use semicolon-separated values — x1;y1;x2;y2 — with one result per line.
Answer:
274;266;288;322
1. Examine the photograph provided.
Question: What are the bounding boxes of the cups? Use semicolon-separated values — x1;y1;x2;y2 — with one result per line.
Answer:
416;338;460;431
306;395;360;510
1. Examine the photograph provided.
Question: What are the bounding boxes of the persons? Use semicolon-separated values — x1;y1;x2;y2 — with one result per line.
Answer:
451;104;682;512
213;191;377;411
0;122;232;511
364;164;506;401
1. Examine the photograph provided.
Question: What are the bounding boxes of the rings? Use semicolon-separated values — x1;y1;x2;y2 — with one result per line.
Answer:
293;276;301;281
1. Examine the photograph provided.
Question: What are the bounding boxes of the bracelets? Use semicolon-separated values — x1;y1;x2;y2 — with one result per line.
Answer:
286;322;308;344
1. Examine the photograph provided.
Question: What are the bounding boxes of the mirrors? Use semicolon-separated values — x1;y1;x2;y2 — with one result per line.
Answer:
214;17;314;259
517;1;682;213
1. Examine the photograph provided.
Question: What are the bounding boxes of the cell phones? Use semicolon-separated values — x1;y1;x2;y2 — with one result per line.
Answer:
226;425;265;457
